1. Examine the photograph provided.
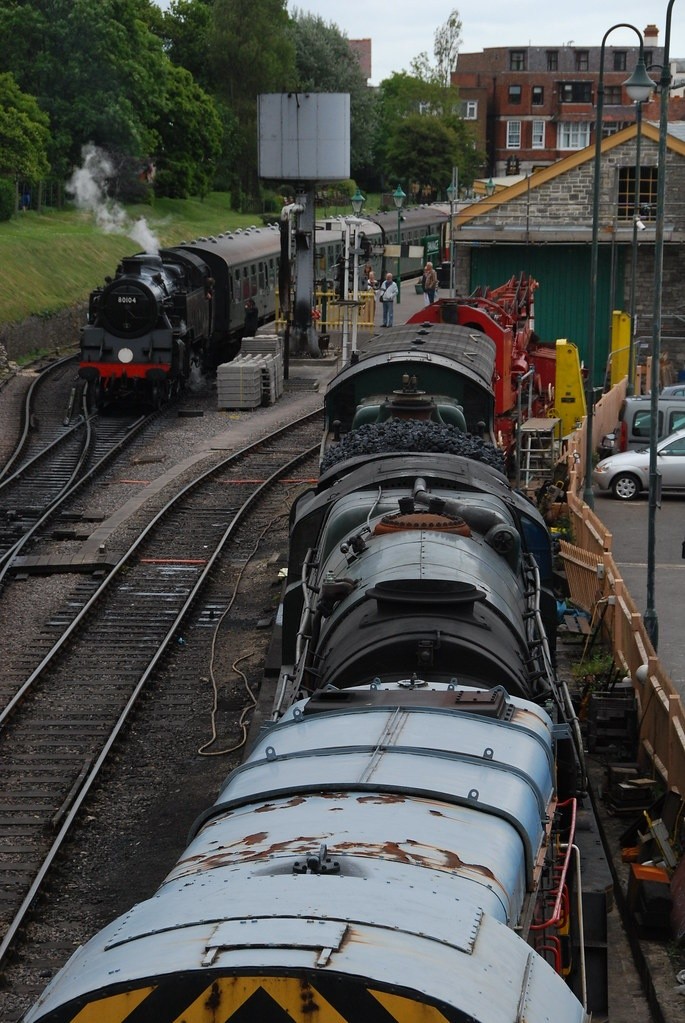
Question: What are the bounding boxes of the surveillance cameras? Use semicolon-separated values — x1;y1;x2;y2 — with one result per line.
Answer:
636;221;646;231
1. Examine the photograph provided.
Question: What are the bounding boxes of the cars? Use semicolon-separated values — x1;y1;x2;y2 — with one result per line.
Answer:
592;427;685;502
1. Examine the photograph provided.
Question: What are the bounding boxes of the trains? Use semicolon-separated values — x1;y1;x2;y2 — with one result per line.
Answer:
76;195;489;414
16;270;601;1023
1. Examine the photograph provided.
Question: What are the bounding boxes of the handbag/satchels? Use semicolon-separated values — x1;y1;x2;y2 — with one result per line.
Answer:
380;296;383;302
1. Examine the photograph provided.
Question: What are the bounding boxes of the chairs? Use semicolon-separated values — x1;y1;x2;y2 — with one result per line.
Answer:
559;590;608;655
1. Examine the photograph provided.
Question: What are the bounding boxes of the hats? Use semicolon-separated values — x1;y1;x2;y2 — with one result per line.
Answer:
247;298;255;306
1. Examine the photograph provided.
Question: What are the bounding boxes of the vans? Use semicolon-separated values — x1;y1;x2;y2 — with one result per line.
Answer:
595;383;685;459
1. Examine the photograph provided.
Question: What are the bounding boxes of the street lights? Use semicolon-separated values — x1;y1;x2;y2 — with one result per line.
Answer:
391;183;406;305
584;25;661;517
446;182;458;262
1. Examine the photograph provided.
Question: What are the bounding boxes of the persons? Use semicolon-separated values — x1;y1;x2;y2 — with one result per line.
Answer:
244;298;258;336
380;272;399;327
367;271;380;297
363;264;371;278
422;266;430;305
425;262;437;303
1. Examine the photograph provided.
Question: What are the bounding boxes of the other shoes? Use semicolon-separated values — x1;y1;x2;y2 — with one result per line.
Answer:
387;324;392;328
380;323;387;327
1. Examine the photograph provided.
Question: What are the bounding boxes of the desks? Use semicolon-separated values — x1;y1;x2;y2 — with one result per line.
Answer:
626;864;673;911
